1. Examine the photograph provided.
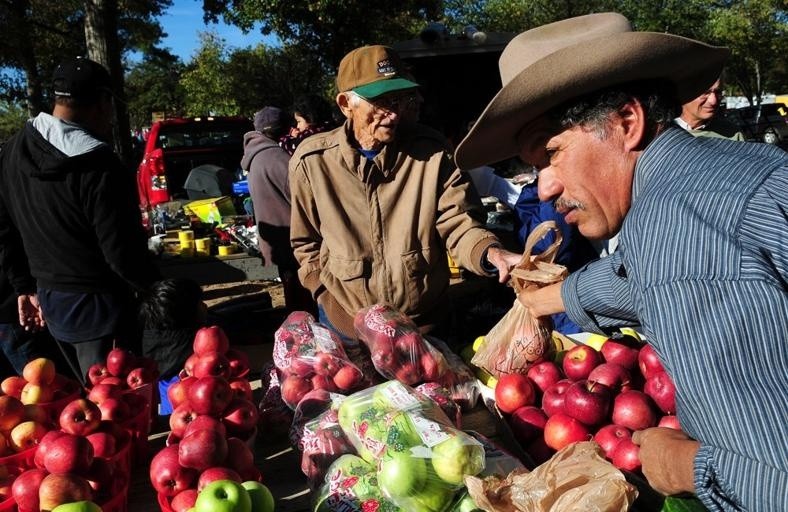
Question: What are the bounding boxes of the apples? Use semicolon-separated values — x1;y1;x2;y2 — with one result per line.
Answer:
51;500;103;512
544;411;589;451
456;495;477;512
309;374;336;392
25;404;52;428
431;433;485;487
38;473;93;512
12;468;50;512
107;347;125;377
402;480;454;512
226;348;250;378
33;430;65;475
599;334;641;370
60;399;102;436
197;467;242;492
313;350;339;377
495;373;536;413
564;379;611;425
170;489;197;512
126;368;144;389
644;371;676;415
184;352;199;377
513;406;547;430
337;455;366;480
86;363;107;384
638;343;665;381
193;325;229;359
187;375;233;415
282;375;313;406
563;344;599;383
23;357;56;386
396;332;423;358
88;383;122;408
0;431;6;456
587;362;631;394
85;432;116;459
585;333;608;351
0;473;17;502
195;479;252;512
240;481;274;512
657;407;682;431
167;375;199;410
169;400;199;439
461;336;498;387
352;472;385;502
394;359;422;386
289;392;352;478
20;382;54;405
528;362;560;393
612;437;642;472
0;395;26;431
8;421;48;454
183;414;226;439
371;342;398;373
378;445;427;498
286;350;314;379
420;350;449;381
228;377;252;400
43;434;94;478
100;376;123;399
193;351;232;381
225;437;253;468
223;400;259;440
150;443;196;497
340;380;455;459
611;390;656;430
274;311;346;368
593;424;632;459
332;362;363;391
541;378;577;418
82;463;109;500
178;428;228;472
355;303;414;343
0;376;28;401
99;398;130;421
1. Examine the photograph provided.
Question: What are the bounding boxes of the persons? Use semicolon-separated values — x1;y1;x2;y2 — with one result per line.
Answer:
0;277;76;379
241;107;314;315
288;44;544;365
279;97;335;157
669;67;747;147
454;11;788;512
0;57;160;386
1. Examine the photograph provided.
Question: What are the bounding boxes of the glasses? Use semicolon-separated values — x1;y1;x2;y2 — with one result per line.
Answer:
699;89;728;100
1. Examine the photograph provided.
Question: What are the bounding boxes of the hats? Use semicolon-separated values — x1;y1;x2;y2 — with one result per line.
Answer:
453;12;730;171
52;56;131;107
336;45;420;101
253;106;290;138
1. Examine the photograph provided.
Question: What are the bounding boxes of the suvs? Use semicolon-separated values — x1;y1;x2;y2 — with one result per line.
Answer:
739;103;788;144
136;118;254;228
